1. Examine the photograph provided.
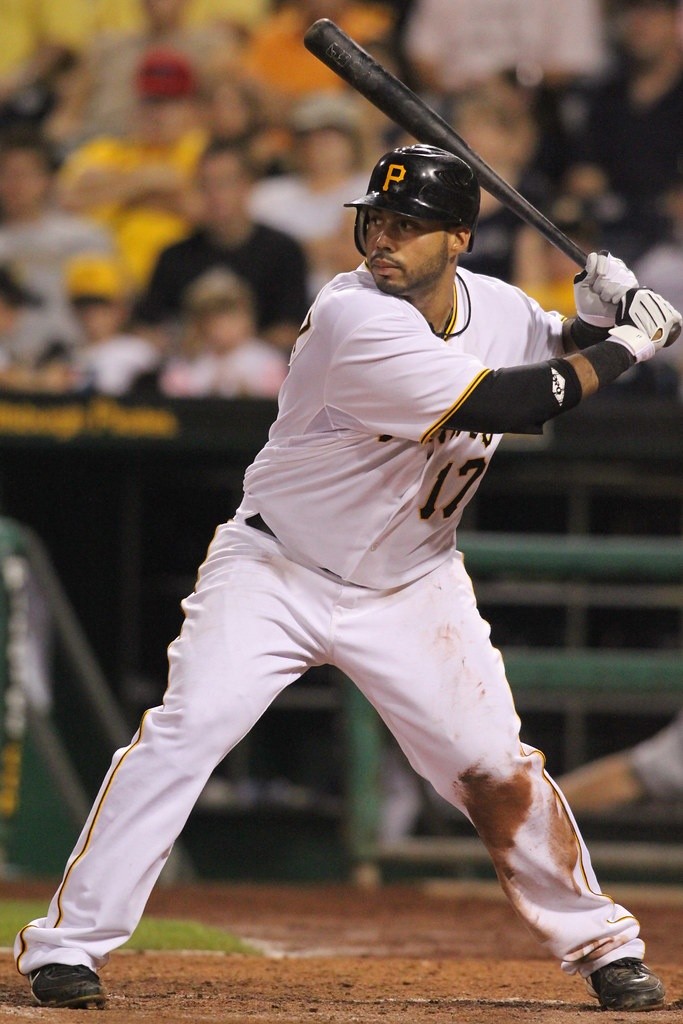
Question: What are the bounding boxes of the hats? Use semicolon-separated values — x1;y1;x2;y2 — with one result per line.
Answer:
60;257;126;307
288;102;363;138
190;267;249;311
132;50;197;100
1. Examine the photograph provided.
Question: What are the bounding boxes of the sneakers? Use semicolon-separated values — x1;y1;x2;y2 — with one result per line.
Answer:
28;962;106;1007
586;957;666;1010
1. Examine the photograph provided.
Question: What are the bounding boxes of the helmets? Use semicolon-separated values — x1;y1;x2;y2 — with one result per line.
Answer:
343;144;481;258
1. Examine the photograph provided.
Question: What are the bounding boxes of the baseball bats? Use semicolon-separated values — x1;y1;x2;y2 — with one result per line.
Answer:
303;17;681;347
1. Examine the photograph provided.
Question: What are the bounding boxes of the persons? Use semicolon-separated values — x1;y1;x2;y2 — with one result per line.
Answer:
0;0;683;403
14;145;681;1006
553;717;683;811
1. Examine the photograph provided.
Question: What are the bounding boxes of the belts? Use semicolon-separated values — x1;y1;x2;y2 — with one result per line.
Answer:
246;513;367;588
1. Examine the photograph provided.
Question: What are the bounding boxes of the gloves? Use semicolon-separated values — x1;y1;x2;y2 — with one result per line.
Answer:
604;286;682;366
570;250;640;335
